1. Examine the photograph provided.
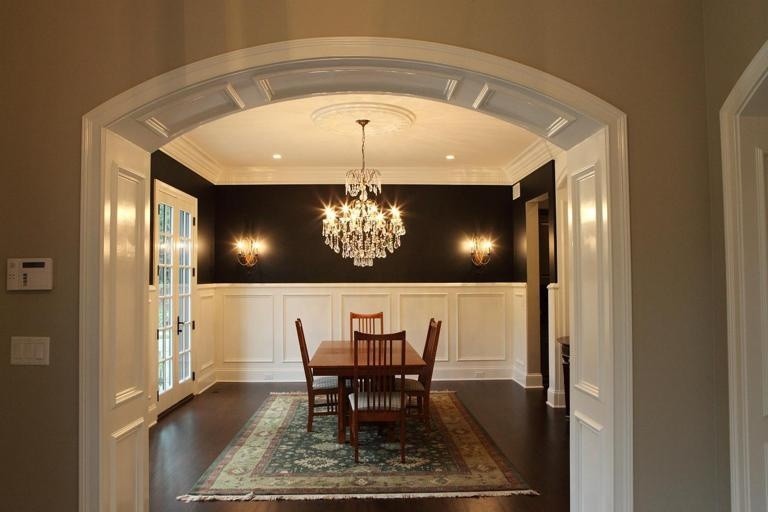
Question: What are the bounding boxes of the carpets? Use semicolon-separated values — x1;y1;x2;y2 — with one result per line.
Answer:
174;388;542;502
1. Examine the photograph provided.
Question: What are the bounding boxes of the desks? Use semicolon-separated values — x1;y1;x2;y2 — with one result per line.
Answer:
308;339;427;443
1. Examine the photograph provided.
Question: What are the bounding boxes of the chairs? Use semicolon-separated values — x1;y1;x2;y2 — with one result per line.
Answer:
347;311;408;462
294;317;446;433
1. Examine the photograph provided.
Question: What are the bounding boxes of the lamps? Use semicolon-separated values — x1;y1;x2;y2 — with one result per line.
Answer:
320;119;406;269
